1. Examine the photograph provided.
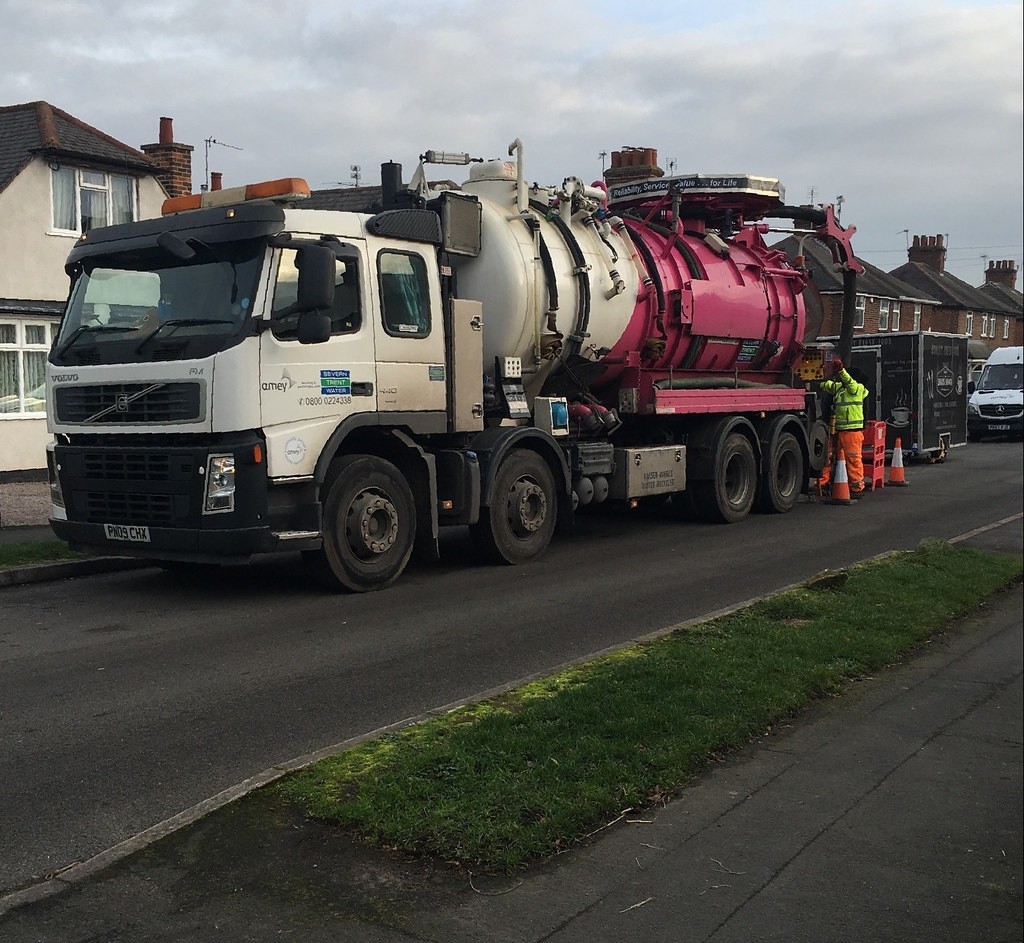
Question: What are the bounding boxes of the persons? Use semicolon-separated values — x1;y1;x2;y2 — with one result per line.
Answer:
819;358;869;500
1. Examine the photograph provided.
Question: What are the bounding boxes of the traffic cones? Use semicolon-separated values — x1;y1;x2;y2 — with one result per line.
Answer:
821;448;858;506
883;436;911;486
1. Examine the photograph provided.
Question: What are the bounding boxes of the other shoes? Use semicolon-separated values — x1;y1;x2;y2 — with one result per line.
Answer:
853;491;866;499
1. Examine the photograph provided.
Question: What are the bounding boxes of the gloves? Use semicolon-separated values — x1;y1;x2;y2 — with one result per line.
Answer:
833;352;844;370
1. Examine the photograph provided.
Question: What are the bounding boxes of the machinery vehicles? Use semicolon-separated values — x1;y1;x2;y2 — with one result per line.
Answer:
42;134;862;595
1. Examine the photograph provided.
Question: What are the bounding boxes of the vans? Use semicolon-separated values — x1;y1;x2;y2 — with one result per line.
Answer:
967;346;1024;442
